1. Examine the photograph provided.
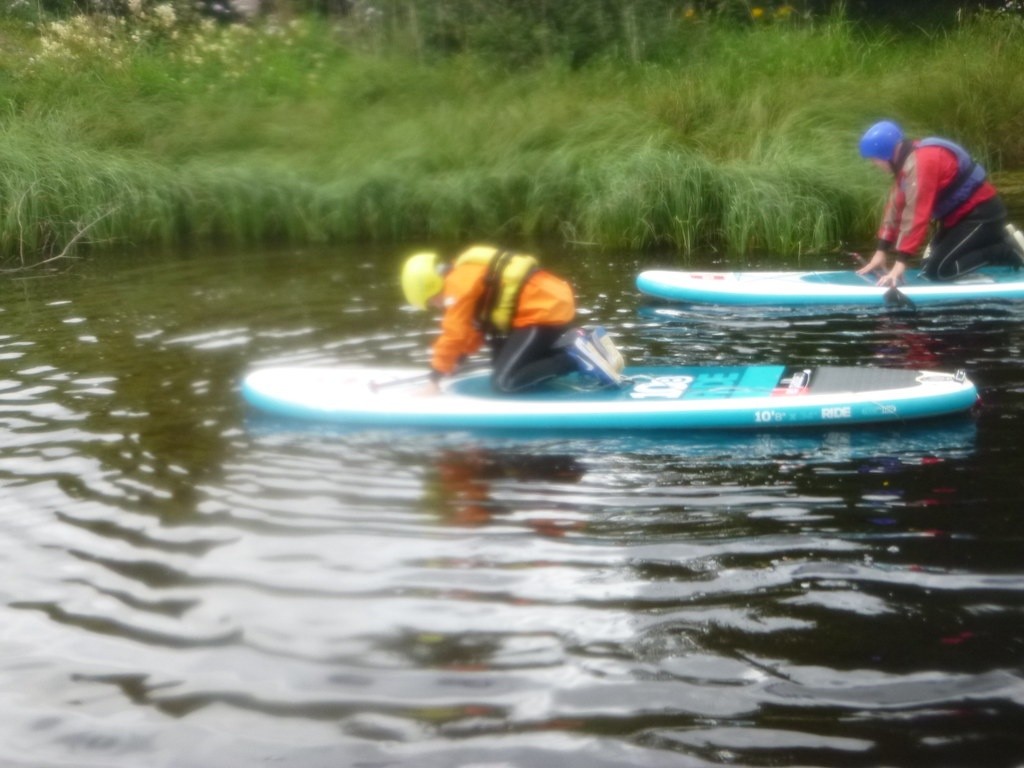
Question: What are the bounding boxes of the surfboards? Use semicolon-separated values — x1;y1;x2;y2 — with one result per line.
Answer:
237;361;982;431
634;261;1024;308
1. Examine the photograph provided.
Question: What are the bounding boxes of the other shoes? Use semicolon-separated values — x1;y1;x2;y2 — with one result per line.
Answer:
565;337;621;387
590;327;625;373
1003;224;1024;261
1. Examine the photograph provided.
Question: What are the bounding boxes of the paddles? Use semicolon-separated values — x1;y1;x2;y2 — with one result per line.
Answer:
367;359;492;396
840;249;917;308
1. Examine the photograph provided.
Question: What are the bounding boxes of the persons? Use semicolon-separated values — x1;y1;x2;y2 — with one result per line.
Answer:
856;120;1024;288
400;245;625;398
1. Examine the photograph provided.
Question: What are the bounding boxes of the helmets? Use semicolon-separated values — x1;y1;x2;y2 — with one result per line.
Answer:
859;121;903;161
401;252;444;310
